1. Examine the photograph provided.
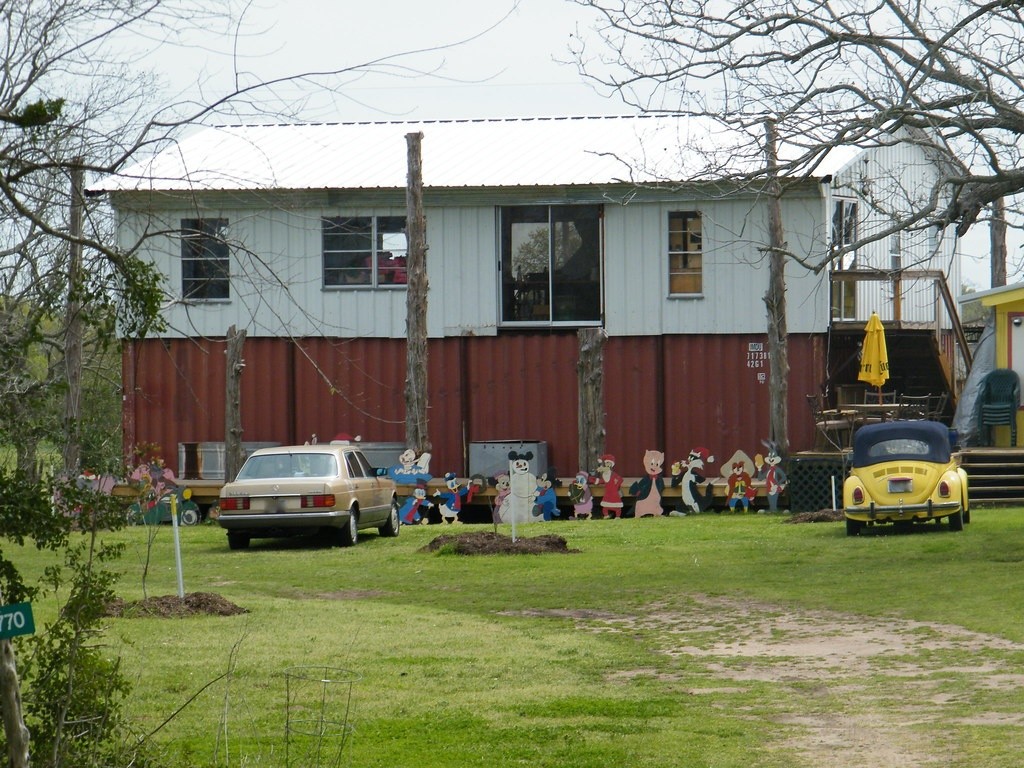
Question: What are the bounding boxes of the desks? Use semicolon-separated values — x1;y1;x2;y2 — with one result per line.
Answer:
838;403;924;449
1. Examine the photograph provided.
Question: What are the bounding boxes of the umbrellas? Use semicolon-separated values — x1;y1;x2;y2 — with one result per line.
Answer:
857;313;890;406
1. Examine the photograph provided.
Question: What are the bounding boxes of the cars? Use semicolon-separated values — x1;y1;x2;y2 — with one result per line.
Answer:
217;444;400;550
843;420;970;536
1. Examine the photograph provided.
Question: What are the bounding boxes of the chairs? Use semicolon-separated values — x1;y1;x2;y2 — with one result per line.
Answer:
892;393;933;424
805;393;857;452
976;368;1020;448
859;390;896;424
929;391;951;422
